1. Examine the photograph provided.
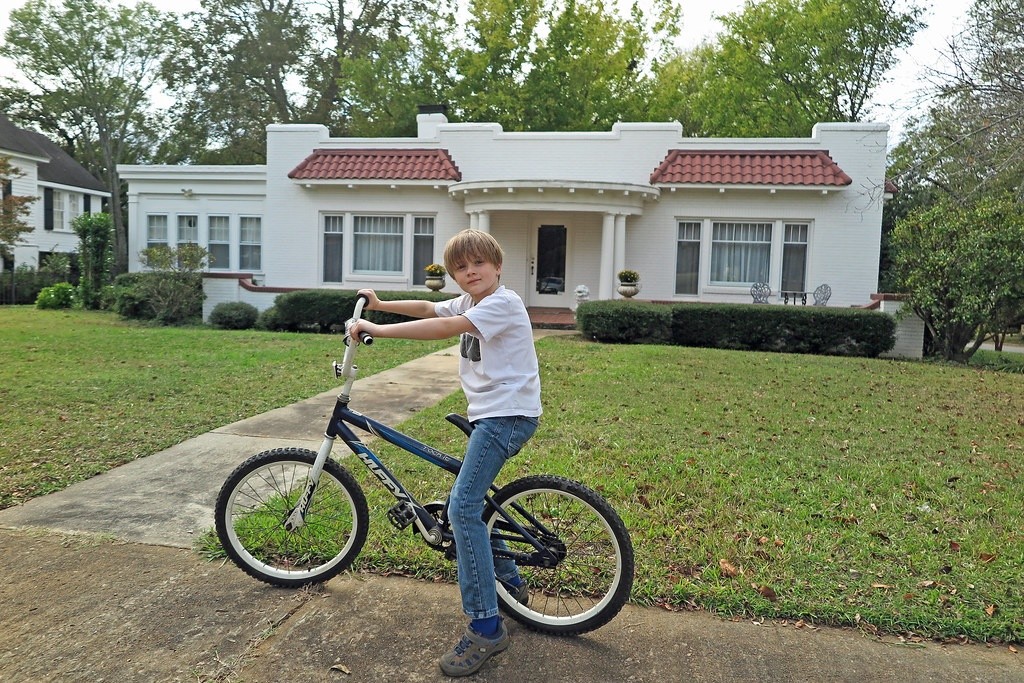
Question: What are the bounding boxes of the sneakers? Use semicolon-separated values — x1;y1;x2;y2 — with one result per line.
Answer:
440;616;509;676
508;583;529;605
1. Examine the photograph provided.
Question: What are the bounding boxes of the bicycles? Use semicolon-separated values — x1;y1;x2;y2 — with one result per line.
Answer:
214;288;635;637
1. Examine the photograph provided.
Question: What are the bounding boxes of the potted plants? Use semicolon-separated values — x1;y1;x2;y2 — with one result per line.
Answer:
616;268;641;300
423;263;447;292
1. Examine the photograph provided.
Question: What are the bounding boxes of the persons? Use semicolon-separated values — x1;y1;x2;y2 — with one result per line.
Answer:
345;228;546;679
